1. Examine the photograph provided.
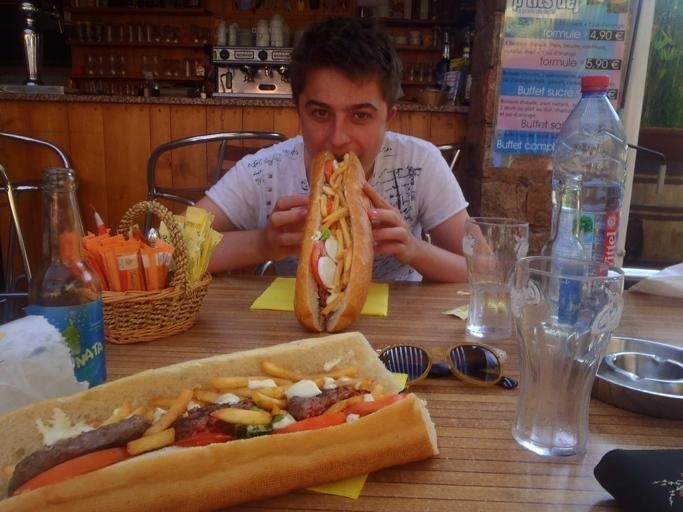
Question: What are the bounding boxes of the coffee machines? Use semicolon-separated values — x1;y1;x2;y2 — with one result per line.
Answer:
209;47;303;100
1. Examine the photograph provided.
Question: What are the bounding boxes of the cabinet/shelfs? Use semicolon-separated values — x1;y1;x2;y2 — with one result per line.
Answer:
63;1;213;98
369;16;460;102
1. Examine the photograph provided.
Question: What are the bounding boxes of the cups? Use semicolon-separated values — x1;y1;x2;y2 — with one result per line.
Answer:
238;28;256;46
388;26;436;48
215;19;236;46
75;81;161;98
73;22;209;46
393;61;432;81
508;250;625;464
78;53;203;79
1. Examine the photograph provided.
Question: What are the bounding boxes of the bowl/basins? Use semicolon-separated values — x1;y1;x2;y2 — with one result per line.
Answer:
256;14;290;47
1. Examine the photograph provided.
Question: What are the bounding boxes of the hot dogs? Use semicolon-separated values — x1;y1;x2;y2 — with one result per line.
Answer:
0;330;439;512
293;150;376;333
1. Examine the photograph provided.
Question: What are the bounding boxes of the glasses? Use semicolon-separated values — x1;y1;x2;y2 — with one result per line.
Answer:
378;342;518;390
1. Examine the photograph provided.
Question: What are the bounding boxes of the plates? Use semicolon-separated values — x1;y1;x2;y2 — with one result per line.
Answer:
591;446;683;512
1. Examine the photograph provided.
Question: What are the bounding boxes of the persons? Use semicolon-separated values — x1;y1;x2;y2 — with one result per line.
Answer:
173;14;502;283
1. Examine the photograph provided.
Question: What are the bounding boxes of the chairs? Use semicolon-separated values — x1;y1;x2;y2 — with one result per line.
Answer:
142;130;289;241
0;129;86;321
421;141;471;245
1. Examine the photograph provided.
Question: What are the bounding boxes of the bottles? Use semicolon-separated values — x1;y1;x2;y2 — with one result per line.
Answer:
548;72;622;271
538;174;586;257
432;31;470;107
26;164;105;386
462;213;529;339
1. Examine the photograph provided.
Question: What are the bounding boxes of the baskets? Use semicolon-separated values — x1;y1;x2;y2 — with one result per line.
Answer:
77;201;213;345
